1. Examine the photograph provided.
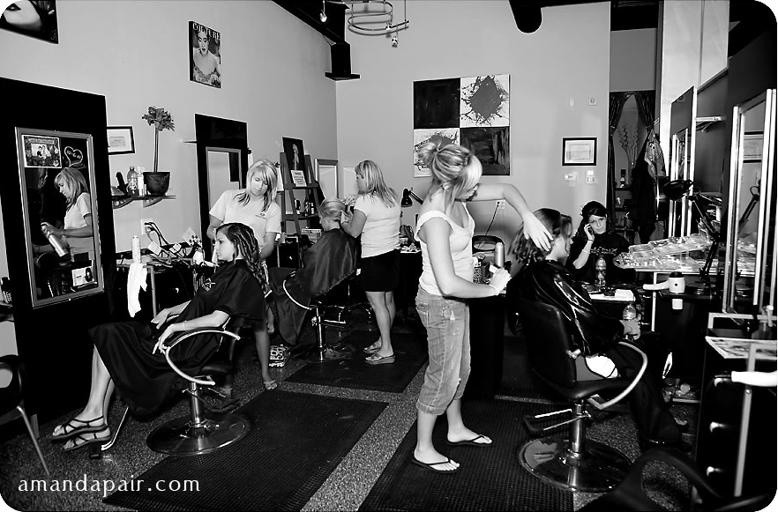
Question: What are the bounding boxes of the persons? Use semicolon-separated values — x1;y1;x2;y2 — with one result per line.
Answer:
4;1;51;35
505;207;697;457
205;158;283;393
409;136;556;473
565;200;637;288
269;197;357;335
48;221;263;453
38;165;96;264
340;159;402;368
36;144;48;157
191;24;221;86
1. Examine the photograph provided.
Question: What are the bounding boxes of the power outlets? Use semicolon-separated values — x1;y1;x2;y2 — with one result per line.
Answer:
141;219;154;233
496;199;505;209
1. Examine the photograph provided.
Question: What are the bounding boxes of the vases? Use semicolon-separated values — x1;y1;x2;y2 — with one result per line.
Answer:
628;161;636;185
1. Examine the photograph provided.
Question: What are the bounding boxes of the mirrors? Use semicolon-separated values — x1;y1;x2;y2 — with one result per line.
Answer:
315;158;338;199
15;127;103;309
205;147;243;211
673;128;688;237
721;90;776;325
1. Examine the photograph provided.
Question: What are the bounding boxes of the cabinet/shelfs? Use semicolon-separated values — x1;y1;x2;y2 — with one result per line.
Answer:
616;187;631;231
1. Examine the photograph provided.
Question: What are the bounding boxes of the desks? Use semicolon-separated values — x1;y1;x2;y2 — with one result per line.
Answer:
636;268;720;331
590;288;635;302
118;259;194;321
267;235;309;268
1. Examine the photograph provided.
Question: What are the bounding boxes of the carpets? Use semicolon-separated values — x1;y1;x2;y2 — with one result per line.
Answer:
357;399;573;512
283;330;429;393
101;388;389;512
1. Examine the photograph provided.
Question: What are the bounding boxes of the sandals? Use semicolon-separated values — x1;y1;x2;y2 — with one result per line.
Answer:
49;416;107;440
60;432;110;452
263;380;277;390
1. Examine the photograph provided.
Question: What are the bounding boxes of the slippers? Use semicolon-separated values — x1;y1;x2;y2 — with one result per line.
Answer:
363;352;395;365
363;343;381;353
411;456;461;474
446;434;492;448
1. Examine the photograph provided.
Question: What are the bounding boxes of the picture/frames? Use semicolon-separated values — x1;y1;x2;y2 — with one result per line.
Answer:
107;125;134;154
743;130;763;163
562;137;597;166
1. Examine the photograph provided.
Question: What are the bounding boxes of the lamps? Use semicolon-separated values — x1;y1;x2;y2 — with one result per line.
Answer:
401;188;424;207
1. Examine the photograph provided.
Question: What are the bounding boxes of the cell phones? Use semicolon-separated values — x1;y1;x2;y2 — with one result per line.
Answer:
587;225;594;235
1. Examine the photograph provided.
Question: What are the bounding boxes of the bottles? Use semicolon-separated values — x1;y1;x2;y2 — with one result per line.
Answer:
127;165;145;196
131;235;142;265
595;255;607;289
494;239;504;267
304;202;315;217
621;302;636;341
39;223;69;258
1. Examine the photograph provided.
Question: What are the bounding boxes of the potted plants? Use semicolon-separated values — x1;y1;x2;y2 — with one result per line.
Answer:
142;107;175;196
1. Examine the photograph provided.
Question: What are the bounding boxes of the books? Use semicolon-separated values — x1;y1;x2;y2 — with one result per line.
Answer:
705;334;776;361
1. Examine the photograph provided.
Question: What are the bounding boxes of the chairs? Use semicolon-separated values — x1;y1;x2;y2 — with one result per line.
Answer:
518;297;648;493
89;288;265;460
0;305;50;476
577;448;765;512
309;268;362;362
38;252;59;298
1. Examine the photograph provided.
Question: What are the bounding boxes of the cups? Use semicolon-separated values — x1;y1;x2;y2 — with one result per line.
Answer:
400;237;407;245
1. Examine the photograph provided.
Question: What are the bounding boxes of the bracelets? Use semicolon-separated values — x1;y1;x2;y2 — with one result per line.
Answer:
581;248;592;256
488;284;499;296
182;320;190;332
586;238;595;244
